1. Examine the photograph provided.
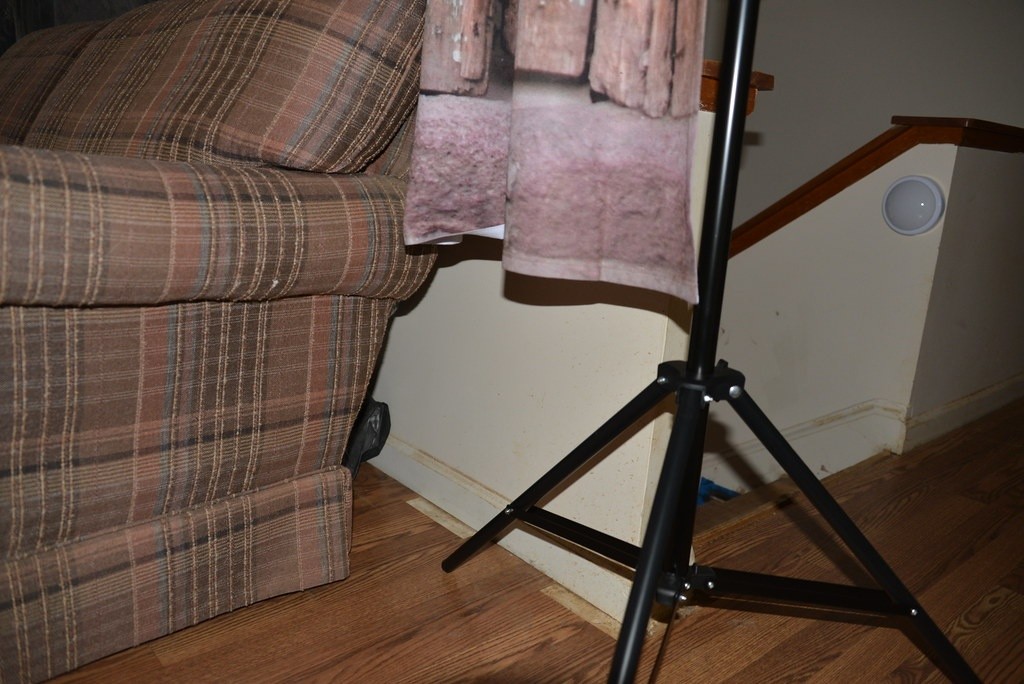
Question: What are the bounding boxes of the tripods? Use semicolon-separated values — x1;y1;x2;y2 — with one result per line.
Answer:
440;0;989;684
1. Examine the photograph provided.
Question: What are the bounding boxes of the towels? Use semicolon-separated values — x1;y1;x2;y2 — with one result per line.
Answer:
403;0;704;310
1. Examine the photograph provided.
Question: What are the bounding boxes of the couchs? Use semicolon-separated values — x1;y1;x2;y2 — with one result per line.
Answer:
0;0;440;684
